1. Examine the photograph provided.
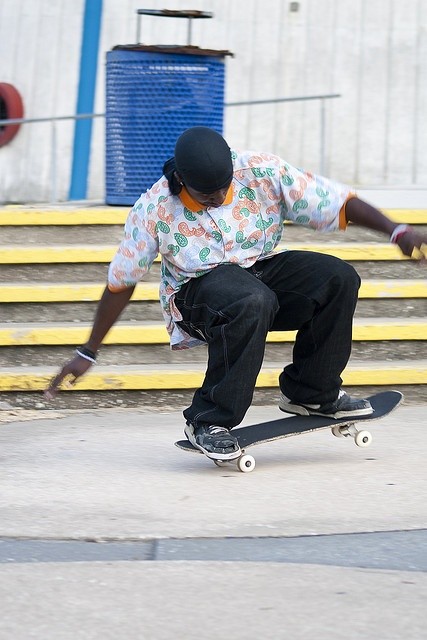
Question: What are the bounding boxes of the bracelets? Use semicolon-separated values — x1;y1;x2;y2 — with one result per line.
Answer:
73;349;97;361
389;224;410;246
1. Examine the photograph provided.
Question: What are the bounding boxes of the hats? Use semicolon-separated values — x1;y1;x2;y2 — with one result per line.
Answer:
163;127;234;196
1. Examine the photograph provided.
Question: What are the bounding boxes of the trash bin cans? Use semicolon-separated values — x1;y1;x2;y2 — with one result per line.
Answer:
105;10;233;206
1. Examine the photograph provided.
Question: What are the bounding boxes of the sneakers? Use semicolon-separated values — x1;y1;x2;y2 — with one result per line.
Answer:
184;420;242;461
278;389;373;419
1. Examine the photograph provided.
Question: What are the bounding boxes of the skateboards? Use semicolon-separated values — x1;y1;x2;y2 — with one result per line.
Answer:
174;388;405;475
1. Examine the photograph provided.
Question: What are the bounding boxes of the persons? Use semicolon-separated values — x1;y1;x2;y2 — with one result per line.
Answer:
43;127;427;460
